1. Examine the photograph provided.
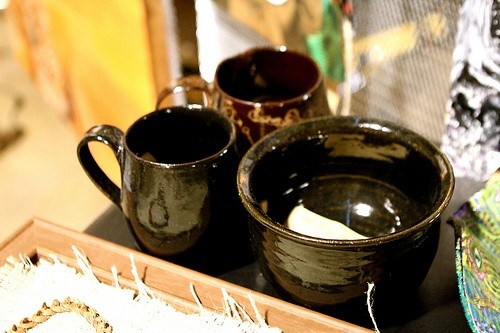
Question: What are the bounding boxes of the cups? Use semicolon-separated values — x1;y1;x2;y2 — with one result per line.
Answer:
77;105;237;255
238;118;456;320
155;48;334;141
